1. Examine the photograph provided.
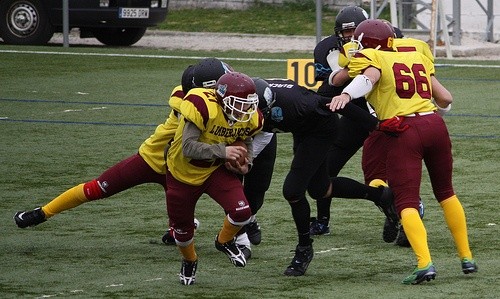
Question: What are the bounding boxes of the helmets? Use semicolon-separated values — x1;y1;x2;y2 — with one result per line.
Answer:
251;77;277;112
335;5;368;47
214;72;257;104
352;19;394;50
181;57;226;93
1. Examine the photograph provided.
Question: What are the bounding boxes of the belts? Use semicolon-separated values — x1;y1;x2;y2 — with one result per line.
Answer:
404;111;434;118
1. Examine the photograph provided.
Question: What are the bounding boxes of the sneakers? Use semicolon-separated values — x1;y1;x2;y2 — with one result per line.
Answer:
233;230;251;260
13;206;48;229
382;203;400;244
402;262;436;285
284;243;314;276
462;258;479;274
180;254;198;286
244;213;262;245
215;233;247;268
162;218;199;246
309;217;331;236
396;202;424;248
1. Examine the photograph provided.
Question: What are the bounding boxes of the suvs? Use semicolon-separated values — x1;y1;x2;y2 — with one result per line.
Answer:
0;0;171;46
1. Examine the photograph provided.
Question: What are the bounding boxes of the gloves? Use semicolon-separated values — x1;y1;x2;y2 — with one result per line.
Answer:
379;116;410;133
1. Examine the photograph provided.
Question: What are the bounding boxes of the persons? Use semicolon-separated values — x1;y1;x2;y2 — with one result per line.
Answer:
192;58;277;259
13;65;196;245
260;5;478;286
165;72;264;286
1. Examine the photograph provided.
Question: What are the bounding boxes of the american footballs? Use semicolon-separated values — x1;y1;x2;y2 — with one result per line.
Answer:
226;140;248;168
